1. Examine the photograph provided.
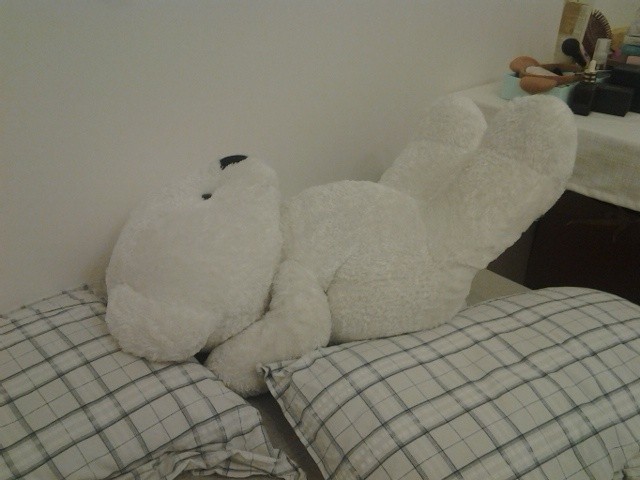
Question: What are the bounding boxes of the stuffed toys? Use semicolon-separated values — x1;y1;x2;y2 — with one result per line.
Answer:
107;95;576;397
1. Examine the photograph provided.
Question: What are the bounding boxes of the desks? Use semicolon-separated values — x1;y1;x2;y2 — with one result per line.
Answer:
453;66;639;306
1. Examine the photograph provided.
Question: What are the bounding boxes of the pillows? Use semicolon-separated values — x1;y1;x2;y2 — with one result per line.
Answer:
1;285;304;480
253;286;640;479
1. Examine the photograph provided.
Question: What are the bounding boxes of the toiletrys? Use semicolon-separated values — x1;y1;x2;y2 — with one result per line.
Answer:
592;38;613;69
572;61;596;116
